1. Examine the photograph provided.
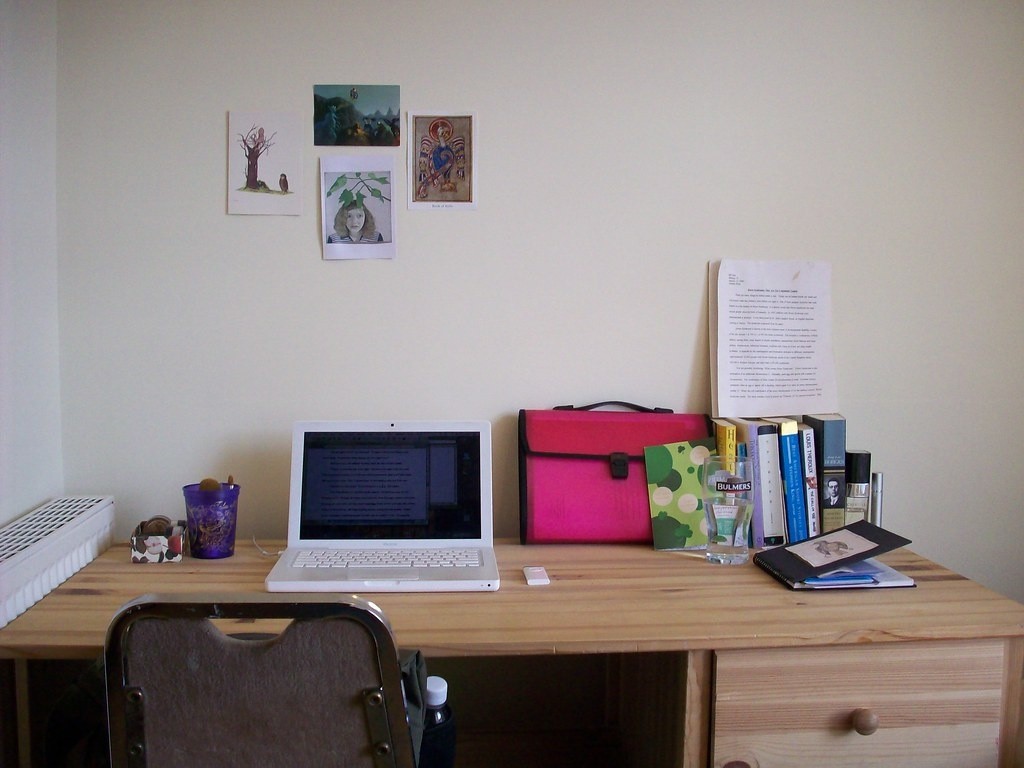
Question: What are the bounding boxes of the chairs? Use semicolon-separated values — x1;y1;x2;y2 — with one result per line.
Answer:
104;590;455;768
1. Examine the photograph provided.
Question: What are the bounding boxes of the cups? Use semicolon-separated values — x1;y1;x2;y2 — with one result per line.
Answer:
183;483;241;560
701;456;753;566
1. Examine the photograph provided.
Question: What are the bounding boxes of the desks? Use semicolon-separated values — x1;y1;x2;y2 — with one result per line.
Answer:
0;534;1024;768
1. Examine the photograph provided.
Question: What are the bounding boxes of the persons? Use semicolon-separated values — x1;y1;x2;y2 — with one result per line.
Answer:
329;199;383;243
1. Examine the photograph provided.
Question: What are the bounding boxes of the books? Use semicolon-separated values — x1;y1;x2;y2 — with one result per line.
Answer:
712;414;915;591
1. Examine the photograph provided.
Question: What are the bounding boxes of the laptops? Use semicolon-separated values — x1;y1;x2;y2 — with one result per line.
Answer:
264;421;500;593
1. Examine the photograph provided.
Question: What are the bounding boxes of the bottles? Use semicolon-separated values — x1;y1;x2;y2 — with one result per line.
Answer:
417;675;456;768
